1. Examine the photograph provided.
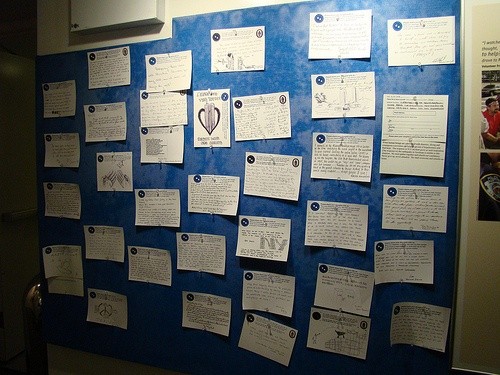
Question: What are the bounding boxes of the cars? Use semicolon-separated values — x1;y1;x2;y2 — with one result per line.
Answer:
482;78;499;97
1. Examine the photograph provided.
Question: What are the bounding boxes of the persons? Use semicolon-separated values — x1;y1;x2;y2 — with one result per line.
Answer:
480;111;489;149
481;98;500;149
